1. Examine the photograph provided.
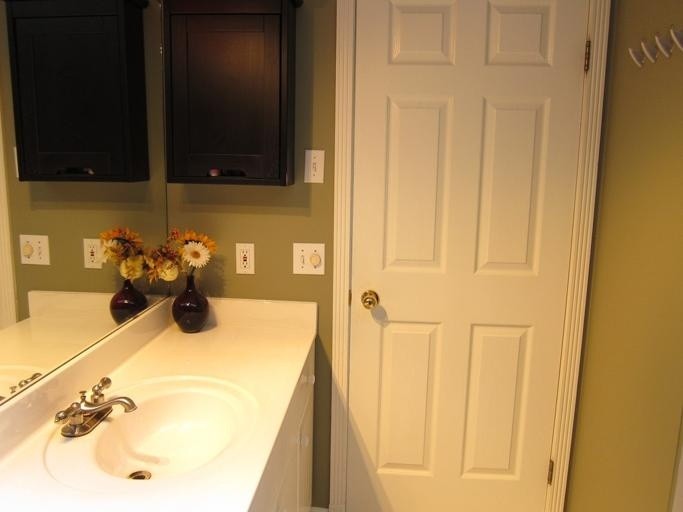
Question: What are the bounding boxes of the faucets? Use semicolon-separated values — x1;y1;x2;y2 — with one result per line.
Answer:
77;397;137;416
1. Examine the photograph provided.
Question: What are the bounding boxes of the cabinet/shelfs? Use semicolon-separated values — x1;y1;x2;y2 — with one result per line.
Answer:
3;0;149;182
165;0;304;186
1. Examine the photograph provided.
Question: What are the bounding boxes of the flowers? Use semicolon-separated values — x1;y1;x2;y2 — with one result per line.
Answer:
147;227;218;285
99;228;153;280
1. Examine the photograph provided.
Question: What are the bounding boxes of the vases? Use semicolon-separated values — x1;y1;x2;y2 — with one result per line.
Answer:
172;275;210;334
110;281;147;328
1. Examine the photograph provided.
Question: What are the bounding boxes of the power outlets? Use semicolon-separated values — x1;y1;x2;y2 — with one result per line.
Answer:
235;243;254;275
84;238;103;269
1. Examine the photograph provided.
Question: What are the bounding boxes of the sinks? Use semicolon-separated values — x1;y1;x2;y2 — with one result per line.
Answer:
94;391;243;479
